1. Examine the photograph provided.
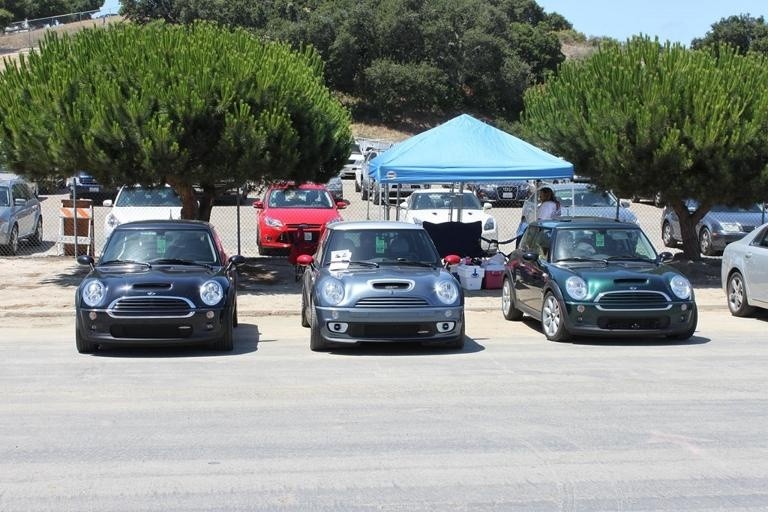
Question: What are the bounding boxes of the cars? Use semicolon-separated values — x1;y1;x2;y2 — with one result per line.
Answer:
516;183;639;252
497;216;698;343
215;176;249;204
294;220;466;351
721;222;768;318
470;180;530;206
0;174;44;254
661;199;767;256
630;189;665;208
254;182;347;253
73;218;246;356
70;170;113;204
400;191;499;251
328;137;430;204
102;182;201;241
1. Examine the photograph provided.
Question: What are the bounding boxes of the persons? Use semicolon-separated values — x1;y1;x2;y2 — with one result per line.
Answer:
536;187;562;223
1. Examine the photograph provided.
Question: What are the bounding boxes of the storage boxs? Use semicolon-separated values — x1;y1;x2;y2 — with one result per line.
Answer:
456;265;484;291
479;263;504;289
